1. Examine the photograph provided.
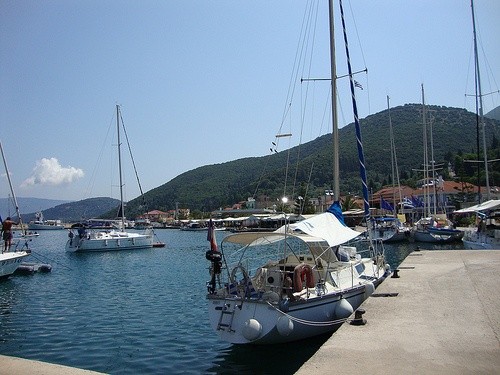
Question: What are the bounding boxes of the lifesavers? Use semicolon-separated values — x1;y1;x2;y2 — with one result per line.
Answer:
293;263;315;292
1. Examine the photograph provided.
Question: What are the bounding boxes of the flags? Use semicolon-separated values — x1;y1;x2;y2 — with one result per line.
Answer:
355;80;364;90
412;196;422;207
207;219;217;251
381;197;393;211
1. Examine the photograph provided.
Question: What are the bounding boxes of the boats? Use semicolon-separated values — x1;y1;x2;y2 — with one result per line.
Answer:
17;231;40;238
341;207;367;229
28;212;64;230
83;213;318;233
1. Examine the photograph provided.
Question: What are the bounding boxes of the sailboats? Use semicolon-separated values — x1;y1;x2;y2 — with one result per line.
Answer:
370;94;411;243
0;140;33;281
452;0;500;250
412;83;465;243
200;0;391;346
65;104;166;252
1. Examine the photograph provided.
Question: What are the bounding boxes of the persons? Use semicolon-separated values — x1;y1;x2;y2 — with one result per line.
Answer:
0;217;20;252
